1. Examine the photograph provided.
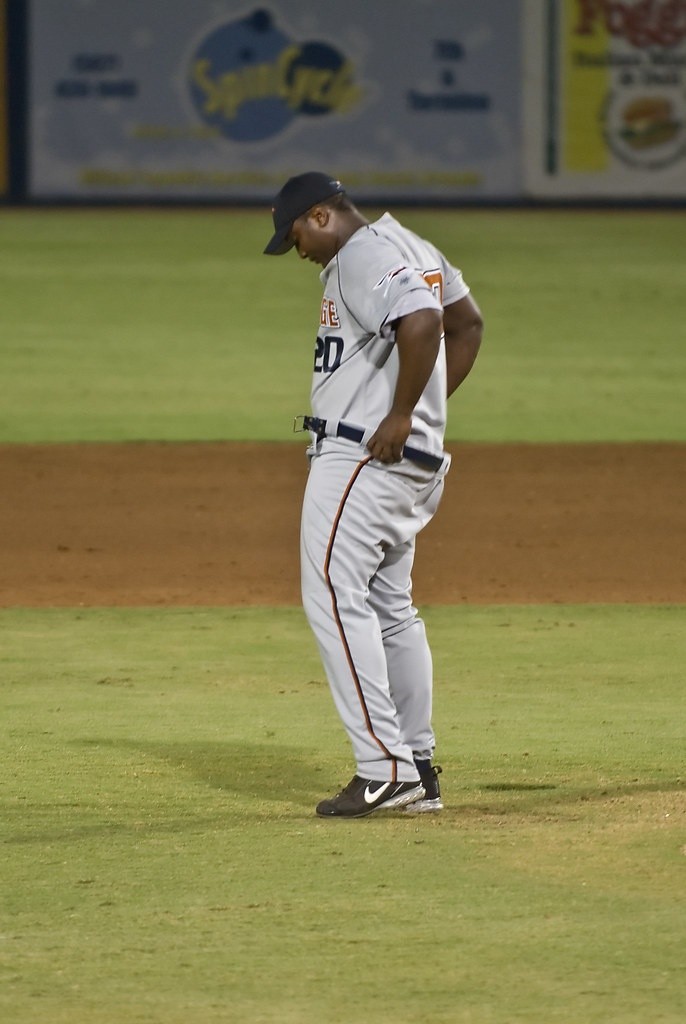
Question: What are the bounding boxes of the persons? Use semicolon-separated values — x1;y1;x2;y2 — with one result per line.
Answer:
261;170;484;819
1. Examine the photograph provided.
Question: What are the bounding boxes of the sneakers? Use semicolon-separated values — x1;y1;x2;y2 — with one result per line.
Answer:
394;765;444;813
316;775;426;819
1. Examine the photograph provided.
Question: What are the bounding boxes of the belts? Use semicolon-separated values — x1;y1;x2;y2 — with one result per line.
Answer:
293;414;448;472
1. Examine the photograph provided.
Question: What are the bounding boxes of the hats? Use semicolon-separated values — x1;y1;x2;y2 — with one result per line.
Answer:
263;172;345;255
